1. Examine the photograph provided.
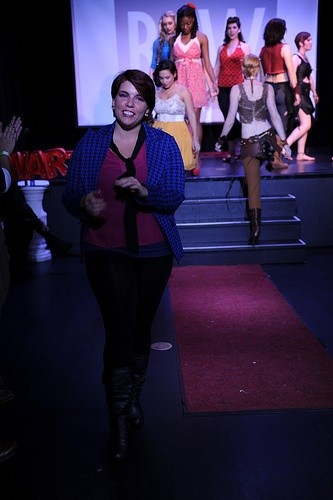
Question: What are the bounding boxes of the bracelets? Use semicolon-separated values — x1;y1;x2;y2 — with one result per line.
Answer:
313;95;318;97
218;135;228;151
280;137;288;147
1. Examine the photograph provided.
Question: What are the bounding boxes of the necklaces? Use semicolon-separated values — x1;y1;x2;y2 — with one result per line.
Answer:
297;51;308;62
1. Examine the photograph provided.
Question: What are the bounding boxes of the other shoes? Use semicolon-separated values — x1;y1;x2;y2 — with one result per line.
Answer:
297;153;315;161
192;160;201;176
282;144;293;160
43;232;72;255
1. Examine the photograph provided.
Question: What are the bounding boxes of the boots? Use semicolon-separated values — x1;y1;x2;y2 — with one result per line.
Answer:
102;369;132;482
129;367;148;428
248;208;261;246
270;137;288;168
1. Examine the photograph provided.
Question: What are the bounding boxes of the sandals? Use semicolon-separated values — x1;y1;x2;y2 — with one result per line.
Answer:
223;154;231;163
234;154;241;160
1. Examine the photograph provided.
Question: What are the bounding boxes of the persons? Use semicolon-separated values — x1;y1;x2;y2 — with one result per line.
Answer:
62;69;185;460
0;116;73;462
281;32;319;161
149;11;177;89
215;54;292;245
151;60;201;170
213;17;252;162
259;18;301;170
171;3;219;175
